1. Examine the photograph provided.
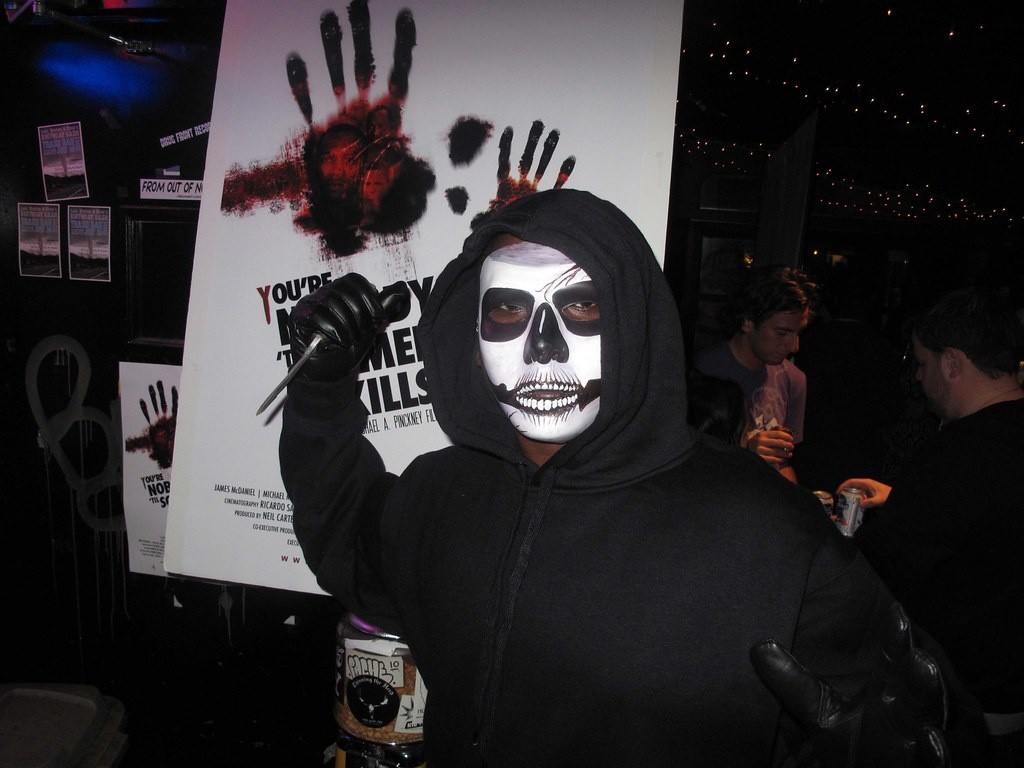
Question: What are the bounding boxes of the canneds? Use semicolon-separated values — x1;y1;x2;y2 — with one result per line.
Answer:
812;490;833;521
835;487;867;536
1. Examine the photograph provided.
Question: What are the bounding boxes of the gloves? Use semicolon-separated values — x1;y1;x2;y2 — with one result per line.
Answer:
751;600;950;768
290;272;411;382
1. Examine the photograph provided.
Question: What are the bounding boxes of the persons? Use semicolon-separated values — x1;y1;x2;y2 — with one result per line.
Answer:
682;264;1024;768
280;188;947;768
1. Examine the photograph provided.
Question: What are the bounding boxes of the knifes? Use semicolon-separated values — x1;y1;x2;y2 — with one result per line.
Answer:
255;335;322;415
